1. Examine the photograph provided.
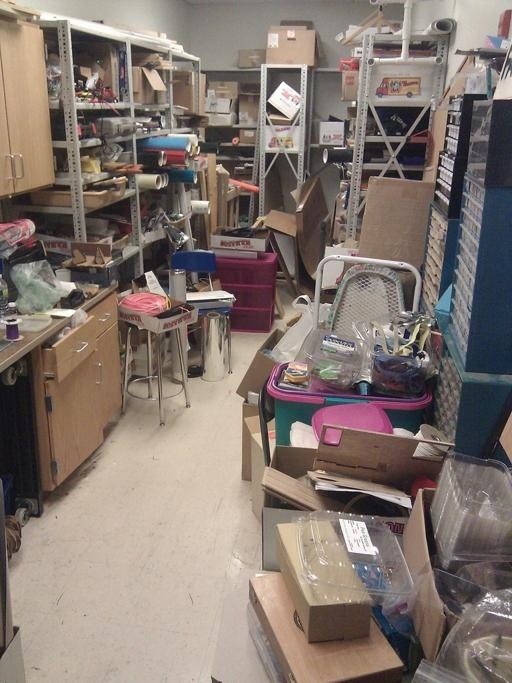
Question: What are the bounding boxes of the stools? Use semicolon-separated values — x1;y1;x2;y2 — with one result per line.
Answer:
121;322;191;428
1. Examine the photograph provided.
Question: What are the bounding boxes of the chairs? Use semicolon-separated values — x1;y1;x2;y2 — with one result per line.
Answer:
169;251;234;374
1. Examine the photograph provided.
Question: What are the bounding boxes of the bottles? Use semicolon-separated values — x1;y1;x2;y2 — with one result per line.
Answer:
0;273;9;325
4;302;19;339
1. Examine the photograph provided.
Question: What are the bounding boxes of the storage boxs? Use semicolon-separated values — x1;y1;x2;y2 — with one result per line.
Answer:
210;252;278;333
173;71;207;115
239;49;265;68
265;27;320;69
131;66;167;105
366;58;434;101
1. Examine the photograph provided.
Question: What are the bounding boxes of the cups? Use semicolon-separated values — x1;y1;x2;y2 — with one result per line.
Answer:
55;268;72;282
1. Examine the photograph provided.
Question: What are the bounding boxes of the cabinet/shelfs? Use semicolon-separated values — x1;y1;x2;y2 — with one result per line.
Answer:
0;13;55;202
32;280;122;493
344;32;450;268
31;19;199;295
414;94;512;459
200;65;359;280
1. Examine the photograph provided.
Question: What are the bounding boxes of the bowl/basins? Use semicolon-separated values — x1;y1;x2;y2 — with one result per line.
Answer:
463;634;511;683
